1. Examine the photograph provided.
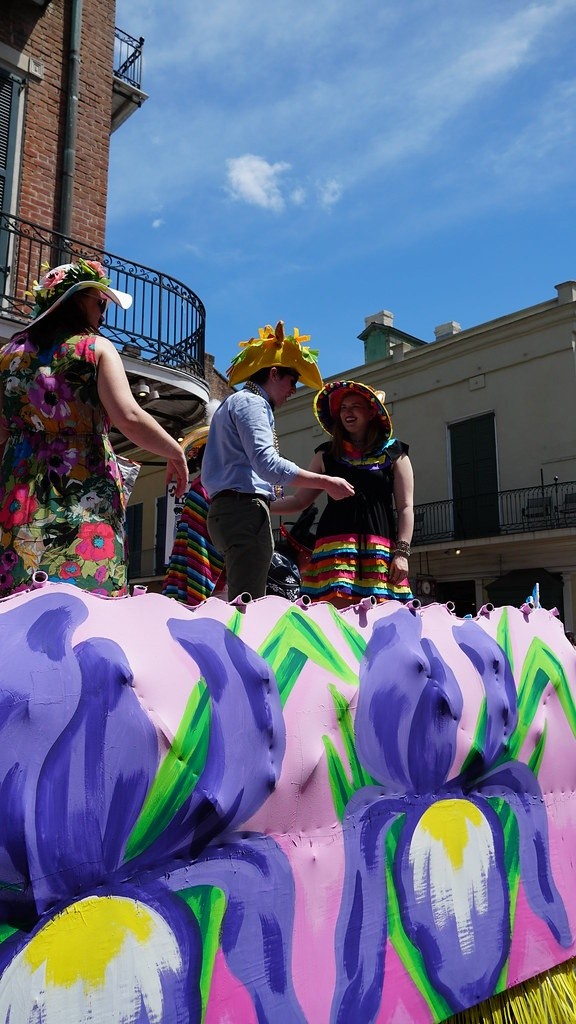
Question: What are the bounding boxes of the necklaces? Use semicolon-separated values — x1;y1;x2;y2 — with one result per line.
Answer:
245;380;281;496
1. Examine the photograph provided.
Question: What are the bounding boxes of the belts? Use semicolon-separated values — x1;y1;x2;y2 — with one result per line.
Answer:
215;489;265;501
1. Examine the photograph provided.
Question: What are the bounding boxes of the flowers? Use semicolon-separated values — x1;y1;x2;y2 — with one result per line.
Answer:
231;323;319;365
24;257;113;320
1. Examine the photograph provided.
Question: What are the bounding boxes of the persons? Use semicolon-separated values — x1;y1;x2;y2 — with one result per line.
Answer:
164;398;228;606
268;380;415;608
201;320;356;603
0;257;191;597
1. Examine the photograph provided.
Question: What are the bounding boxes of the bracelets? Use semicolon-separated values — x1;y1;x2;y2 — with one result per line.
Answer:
394;541;411;559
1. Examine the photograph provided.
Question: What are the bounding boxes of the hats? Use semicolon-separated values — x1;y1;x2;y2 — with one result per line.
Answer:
21;258;133;332
226;320;323;391
180;426;210;461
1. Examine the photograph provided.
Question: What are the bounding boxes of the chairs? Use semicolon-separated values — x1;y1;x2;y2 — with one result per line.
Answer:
559;493;576;528
414;512;425;545
521;496;555;531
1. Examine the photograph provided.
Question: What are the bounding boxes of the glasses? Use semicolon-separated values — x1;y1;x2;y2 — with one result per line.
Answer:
313;380;393;441
84;293;107;314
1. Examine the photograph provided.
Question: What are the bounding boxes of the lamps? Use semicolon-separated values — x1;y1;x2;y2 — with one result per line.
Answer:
455;548;461;555
135;379;159;402
175;430;185;442
